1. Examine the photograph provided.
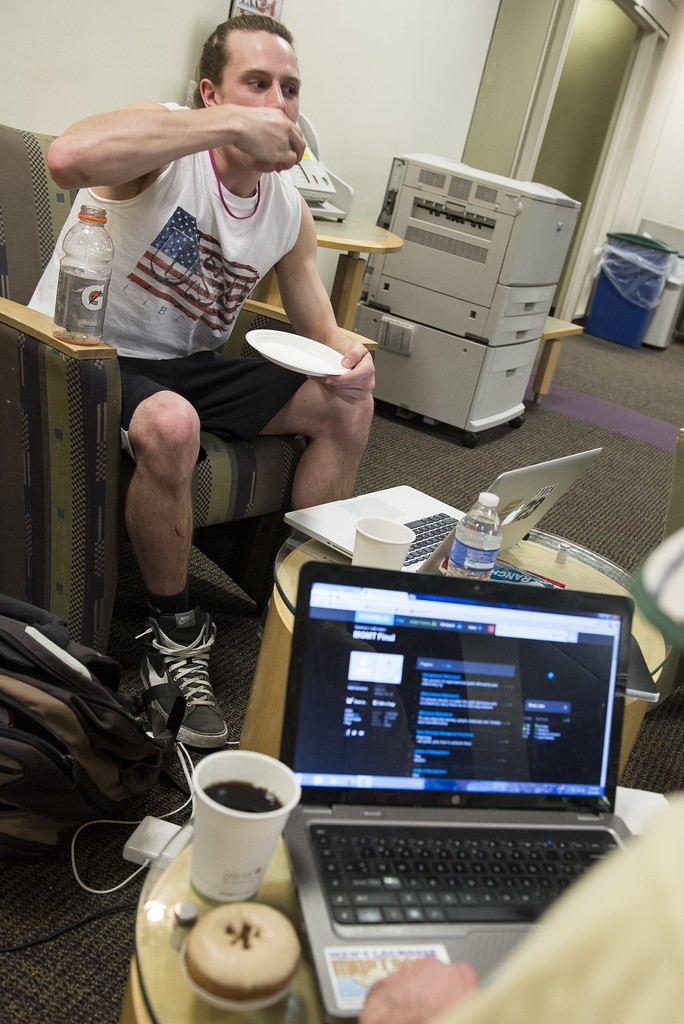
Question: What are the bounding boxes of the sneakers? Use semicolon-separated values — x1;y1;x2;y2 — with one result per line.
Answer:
135;600;229;748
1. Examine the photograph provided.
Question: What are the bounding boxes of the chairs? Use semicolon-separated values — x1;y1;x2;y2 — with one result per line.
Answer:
0;126;379;646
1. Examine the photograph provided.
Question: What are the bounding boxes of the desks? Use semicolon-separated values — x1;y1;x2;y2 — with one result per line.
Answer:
238;527;676;786
254;212;406;335
119;787;356;1024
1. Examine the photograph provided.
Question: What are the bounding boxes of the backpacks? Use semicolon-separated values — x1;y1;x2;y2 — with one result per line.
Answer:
0;594;188;860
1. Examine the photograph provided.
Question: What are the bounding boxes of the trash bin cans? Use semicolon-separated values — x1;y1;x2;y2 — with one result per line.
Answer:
582;231;684;350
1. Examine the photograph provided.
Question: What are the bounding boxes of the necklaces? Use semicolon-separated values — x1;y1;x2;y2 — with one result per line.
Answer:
208;148;261;220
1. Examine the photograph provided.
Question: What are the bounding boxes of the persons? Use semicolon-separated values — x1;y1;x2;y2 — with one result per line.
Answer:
26;14;376;747
359;791;683;1023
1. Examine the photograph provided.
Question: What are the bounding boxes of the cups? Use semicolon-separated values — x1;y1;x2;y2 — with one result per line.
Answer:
191;751;301;904
348;518;414;568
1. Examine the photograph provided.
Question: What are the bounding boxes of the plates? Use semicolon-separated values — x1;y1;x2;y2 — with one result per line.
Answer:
246;328;349;377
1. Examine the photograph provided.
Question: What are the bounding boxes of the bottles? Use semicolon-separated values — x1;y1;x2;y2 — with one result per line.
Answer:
55;203;114;340
447;491;502;577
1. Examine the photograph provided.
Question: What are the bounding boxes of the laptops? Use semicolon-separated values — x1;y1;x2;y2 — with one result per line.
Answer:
283;446;603;574
281;560;634;1024
